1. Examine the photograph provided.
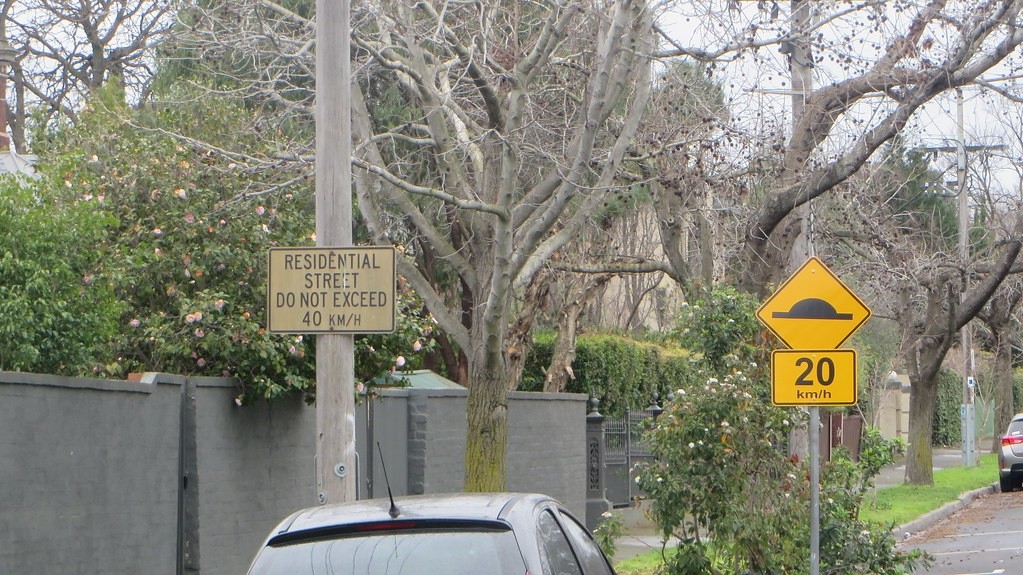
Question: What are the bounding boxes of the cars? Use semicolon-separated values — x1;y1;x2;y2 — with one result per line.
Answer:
244;492;619;575
997;412;1022;493
